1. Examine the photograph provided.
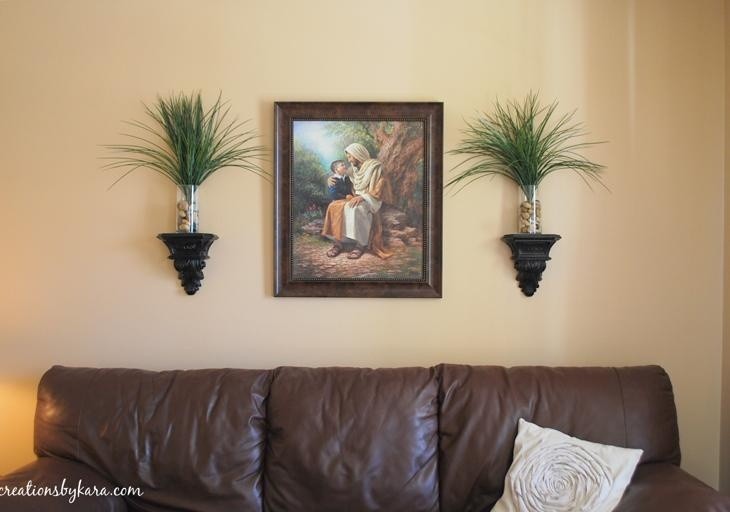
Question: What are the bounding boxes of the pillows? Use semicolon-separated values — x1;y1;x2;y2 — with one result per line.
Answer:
485;412;645;511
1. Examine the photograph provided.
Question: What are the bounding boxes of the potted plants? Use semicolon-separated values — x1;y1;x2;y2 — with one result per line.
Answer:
442;83;616;235
97;87;273;233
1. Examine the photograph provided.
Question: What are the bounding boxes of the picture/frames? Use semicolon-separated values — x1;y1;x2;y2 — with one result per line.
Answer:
271;98;445;300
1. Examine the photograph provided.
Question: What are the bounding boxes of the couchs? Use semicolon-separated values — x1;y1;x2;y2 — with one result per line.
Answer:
4;366;726;509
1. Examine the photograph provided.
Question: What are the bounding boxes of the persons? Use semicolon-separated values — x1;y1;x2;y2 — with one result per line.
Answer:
327;158;358;200
321;141;398;260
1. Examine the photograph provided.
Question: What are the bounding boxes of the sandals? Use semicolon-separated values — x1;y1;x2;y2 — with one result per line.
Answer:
347;247;364;259
327;245;344;257
